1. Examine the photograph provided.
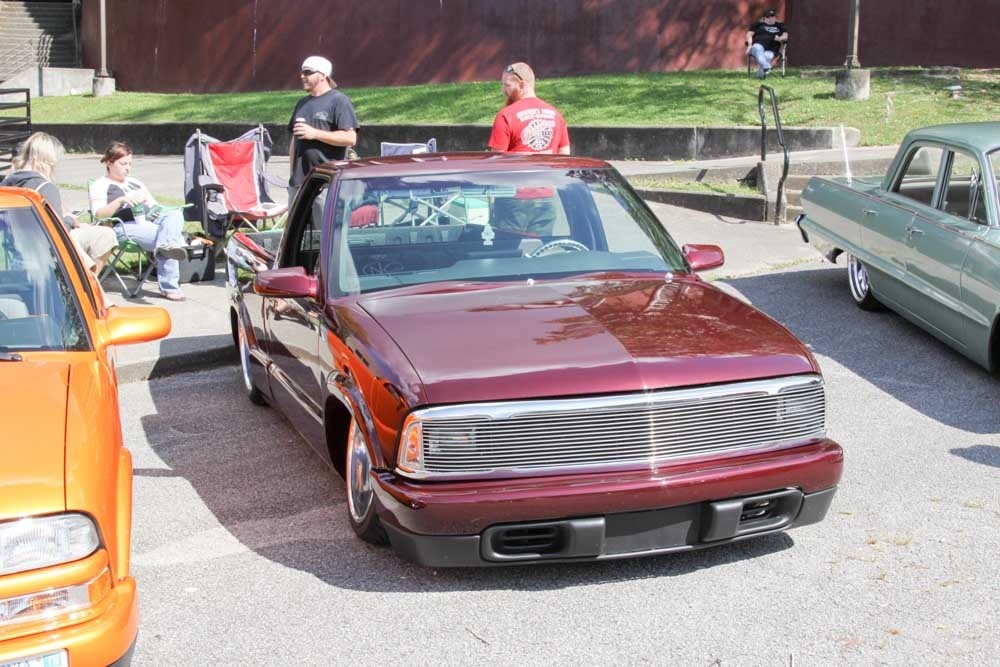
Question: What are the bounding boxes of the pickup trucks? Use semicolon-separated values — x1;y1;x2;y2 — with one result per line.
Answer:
224;150;844;576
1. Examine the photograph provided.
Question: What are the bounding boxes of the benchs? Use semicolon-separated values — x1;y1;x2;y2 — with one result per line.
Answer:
899;178;989;215
297;235;580;279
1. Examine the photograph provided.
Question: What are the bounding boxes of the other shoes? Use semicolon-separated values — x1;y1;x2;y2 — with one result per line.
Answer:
764;68;771;74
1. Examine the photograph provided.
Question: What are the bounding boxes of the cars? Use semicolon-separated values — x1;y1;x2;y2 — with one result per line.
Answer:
796;121;1000;375
0;182;173;667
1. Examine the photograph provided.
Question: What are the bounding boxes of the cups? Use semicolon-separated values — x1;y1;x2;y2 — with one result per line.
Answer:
294;117;306;137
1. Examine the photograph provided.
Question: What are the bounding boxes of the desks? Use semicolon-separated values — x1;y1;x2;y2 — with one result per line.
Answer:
89;180;172;300
376;139;465;228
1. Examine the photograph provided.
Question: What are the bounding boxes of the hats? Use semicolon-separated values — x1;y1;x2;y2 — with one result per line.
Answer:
302;56;337;88
765;10;775;17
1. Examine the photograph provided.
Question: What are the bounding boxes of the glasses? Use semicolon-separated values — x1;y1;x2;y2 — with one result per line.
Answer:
301;70;315;76
506;65;522;80
767;14;775;18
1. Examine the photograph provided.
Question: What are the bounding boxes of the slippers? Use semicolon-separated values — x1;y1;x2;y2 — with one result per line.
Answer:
161;292;185;302
158;247;187;259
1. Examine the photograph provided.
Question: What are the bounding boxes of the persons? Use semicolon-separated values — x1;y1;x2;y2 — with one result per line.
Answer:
488;61;571;237
88;139;188;300
0;131;120;280
286;53;360;229
745;9;788;79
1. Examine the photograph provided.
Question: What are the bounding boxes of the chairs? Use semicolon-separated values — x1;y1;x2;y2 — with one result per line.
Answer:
745;41;788;78
183;125;288;268
